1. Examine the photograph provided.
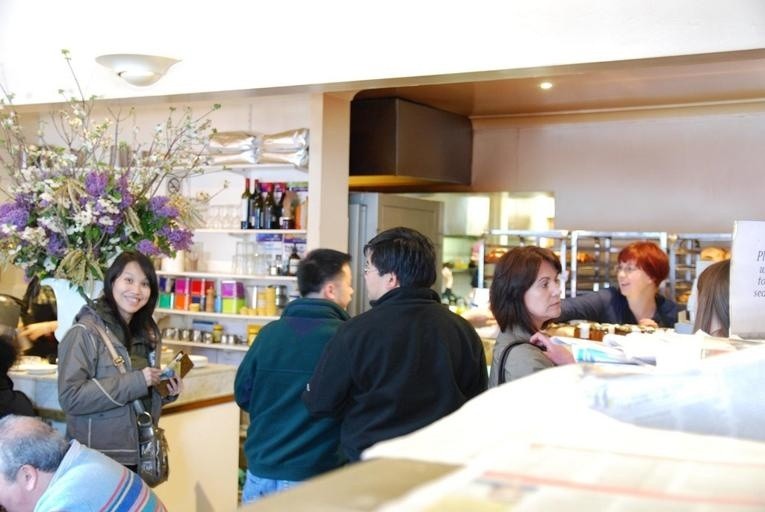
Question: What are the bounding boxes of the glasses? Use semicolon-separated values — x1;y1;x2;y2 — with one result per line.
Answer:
615;261;642;272
361;267;380;274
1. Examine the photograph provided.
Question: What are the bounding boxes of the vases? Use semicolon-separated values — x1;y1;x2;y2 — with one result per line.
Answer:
38;274;104;350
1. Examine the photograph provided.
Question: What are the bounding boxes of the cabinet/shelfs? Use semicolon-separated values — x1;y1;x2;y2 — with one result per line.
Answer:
0;96;311;353
475;229;731;312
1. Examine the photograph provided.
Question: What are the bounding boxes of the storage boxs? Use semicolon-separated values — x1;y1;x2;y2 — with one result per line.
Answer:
353;100;473;183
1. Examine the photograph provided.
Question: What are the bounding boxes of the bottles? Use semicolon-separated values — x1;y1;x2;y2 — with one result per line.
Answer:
205;285;215;312
240;178;251;229
278;188;294;229
287;242;300;276
262;183;277;229
249;179;262;229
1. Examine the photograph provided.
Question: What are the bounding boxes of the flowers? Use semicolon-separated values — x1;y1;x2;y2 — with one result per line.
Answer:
1;100;216;303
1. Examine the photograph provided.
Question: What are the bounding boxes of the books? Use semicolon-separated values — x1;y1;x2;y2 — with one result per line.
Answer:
154;349;195;398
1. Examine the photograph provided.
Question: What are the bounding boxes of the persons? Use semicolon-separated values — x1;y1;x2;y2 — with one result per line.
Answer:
300;225;488;464
57;250;184;487
1;414;170;512
17;275;58;363
694;259;730;338
490;245;575;387
233;249;355;504
557;241;691;329
0;334;37;417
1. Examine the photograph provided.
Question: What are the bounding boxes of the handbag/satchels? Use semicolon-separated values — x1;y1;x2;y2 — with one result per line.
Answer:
88;319;169;487
